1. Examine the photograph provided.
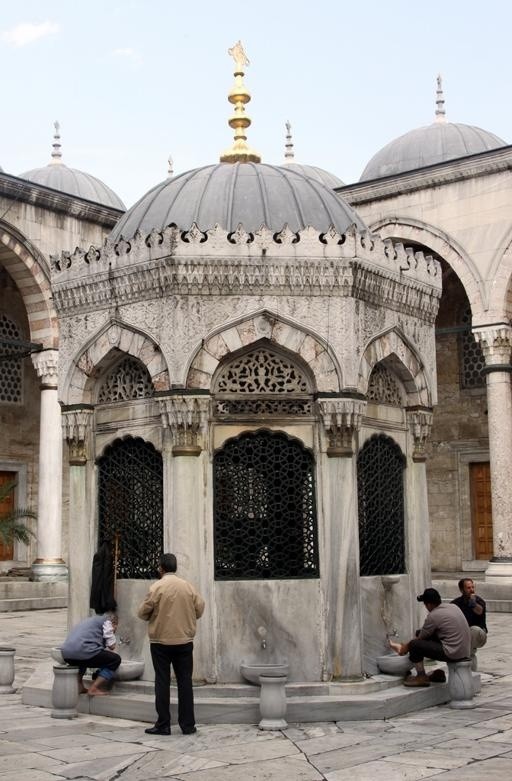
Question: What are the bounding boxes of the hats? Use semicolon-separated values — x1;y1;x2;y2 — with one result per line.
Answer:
416;587;441;602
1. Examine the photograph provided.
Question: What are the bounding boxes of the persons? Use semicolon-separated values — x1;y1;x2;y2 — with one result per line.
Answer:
388;588;471;686
137;552;206;735
60;611;122;696
416;578;488;647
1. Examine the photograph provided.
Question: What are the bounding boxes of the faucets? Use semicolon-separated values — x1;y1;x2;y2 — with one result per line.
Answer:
386;629;401;638
261;640;267;649
117;636;130;645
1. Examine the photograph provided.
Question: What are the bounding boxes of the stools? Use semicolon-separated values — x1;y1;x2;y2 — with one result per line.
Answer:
0;646;19;694
49;664;79;717
447;656;479;709
256;671;288;730
469;647;478;672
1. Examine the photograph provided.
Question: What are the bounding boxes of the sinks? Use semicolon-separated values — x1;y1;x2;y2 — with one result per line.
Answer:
51;647;66;665
239;663;290;685
111;661;144;681
376;651;416;675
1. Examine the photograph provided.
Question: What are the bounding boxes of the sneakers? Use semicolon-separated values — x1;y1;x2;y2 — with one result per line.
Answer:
428;669;446;683
404;675;431;688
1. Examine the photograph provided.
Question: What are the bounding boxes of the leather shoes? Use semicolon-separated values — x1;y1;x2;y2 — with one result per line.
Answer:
144;724;171;736
182;726;197;734
